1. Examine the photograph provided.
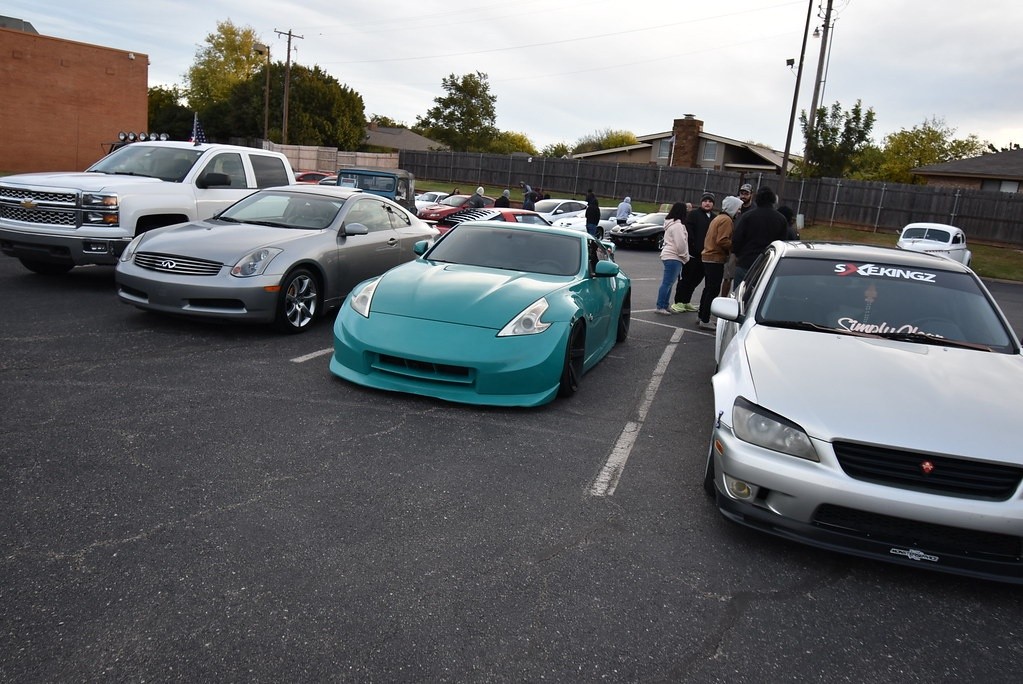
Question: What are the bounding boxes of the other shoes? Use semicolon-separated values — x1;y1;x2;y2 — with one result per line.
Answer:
655;309;671;315
699;320;716;330
665;305;678;312
696;318;716;325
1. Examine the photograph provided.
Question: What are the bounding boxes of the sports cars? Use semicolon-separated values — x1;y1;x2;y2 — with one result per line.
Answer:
328;219;632;409
609;211;670;251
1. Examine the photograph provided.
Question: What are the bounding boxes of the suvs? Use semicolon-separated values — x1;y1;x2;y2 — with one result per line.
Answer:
0;131;296;277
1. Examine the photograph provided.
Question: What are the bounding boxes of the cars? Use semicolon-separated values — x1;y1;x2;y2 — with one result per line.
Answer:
704;238;1022;588
114;185;443;335
893;222;972;272
412;190;644;240
294;170;341;185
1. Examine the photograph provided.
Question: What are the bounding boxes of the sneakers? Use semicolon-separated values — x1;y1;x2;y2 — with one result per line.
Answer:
671;302;686;312
683;303;698;312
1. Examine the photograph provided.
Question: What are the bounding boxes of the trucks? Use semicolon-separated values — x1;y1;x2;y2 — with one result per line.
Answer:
336;166;418;217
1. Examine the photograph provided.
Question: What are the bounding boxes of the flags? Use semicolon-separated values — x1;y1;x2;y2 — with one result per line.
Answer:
188;116;207;143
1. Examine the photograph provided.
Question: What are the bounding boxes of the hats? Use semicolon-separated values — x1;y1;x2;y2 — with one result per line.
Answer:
700;192;715;203
739;184;752;193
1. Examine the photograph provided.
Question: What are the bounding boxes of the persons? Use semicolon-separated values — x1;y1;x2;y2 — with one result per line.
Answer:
522;191;538;224
670;192;718;313
451;188;460;195
469;187;485;208
696;196;745;331
585;189;595;201
585;199;601;236
655;202;690;315
519;181;532;196
494;189;510;208
616;197;632;224
721;184;800;298
